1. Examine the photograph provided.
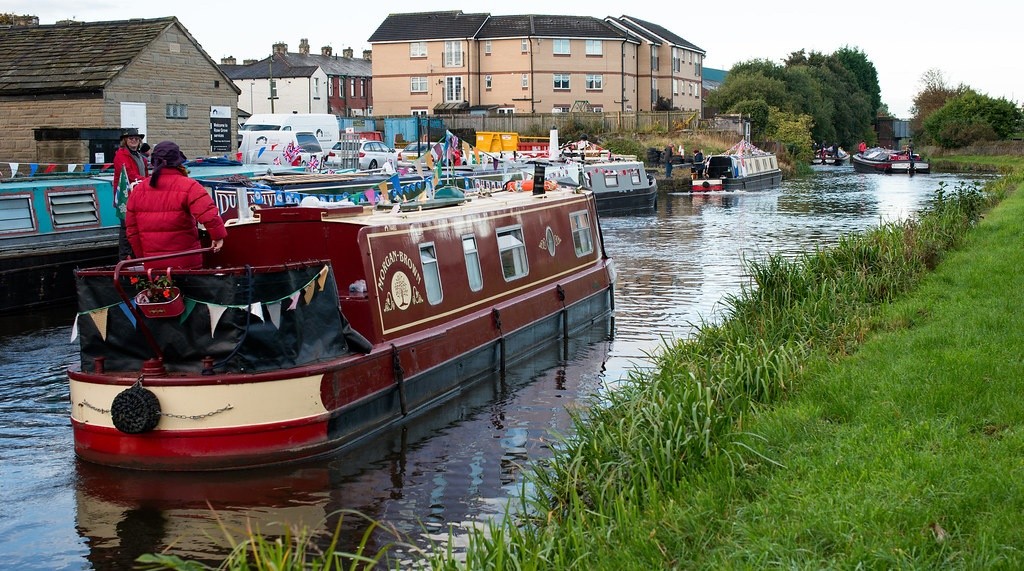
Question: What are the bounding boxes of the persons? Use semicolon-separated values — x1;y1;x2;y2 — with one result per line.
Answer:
836;146;847;158
908;139;915;154
859;140;867;154
665;143;675;178
140;142;151;177
126;140;227;279
113;127;149;263
692;150;705;179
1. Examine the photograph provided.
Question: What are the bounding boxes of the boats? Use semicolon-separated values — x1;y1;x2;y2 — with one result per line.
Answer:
852;146;930;175
812;148;851;166
0;148;659;326
688;135;782;192
60;169;620;476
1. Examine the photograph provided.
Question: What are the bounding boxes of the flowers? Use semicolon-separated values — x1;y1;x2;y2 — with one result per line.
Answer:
128;274;176;302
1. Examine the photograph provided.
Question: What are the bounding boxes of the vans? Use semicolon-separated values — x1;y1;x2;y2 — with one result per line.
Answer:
237;129;326;167
240;113;340;155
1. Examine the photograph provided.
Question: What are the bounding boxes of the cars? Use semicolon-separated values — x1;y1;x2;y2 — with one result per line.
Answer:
396;141;449;171
322;139;398;172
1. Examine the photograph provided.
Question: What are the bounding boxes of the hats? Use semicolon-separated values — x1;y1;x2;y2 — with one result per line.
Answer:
119;129;145;141
151;141;186;172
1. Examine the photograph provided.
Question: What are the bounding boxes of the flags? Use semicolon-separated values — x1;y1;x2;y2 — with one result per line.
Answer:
307;157;318;171
328;169;335;174
270;145;302;165
